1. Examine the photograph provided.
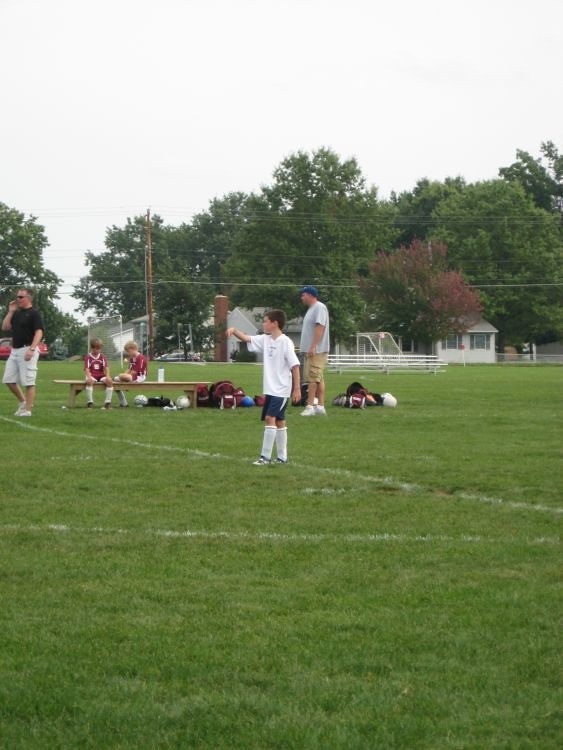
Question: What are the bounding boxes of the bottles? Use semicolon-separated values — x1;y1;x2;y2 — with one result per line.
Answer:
158;366;164;383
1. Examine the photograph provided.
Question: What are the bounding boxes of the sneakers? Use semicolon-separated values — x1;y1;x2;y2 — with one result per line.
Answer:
15;401;31;417
270;457;288;465
300;408;314;416
314;405;327;415
253;456;271;466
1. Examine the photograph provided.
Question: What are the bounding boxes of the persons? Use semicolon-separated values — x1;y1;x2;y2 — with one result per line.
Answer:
83;338;113;409
296;286;330;416
2;288;45;416
224;310;302;466
113;341;147;408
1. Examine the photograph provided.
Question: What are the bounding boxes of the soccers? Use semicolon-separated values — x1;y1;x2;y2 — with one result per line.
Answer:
176;395;190;409
133;394;148;407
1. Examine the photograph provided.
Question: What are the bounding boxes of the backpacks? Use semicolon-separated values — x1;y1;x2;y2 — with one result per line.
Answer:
333;383;382;409
197;381;245;407
293;383;309;406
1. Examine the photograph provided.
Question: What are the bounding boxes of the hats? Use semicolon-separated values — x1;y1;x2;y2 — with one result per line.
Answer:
298;286;319;297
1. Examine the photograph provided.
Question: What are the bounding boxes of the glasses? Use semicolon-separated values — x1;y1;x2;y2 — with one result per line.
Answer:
17;295;30;299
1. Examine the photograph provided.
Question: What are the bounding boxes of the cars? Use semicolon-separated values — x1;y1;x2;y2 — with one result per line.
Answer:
154;352;201;362
0;338;48;359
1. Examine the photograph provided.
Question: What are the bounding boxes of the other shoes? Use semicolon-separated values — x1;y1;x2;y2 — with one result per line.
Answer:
88;402;94;408
120;404;130;408
105;402;112;409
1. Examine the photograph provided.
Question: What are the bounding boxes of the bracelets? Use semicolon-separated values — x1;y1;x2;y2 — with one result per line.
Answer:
29;347;35;352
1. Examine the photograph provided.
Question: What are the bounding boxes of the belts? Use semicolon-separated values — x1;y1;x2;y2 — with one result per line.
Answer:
14;343;31;348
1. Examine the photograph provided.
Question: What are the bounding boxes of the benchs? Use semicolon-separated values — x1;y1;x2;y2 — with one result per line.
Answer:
53;380;214;408
327;354;449;377
